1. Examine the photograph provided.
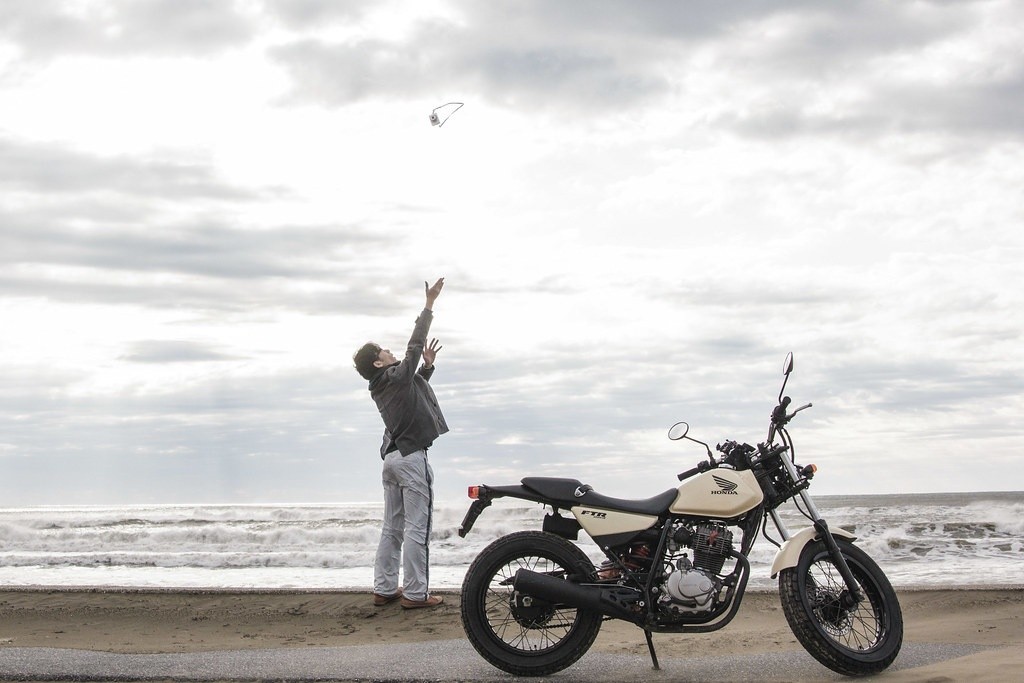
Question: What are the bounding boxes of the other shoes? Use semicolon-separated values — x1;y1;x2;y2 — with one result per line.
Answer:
401;595;444;609
373;587;403;606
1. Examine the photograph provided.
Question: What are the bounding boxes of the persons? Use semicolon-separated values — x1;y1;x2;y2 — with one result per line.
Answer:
353;277;449;610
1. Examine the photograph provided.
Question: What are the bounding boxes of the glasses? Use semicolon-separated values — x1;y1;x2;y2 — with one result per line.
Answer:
374;348;382;357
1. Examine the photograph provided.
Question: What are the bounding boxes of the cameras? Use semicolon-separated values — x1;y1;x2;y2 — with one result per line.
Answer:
428;112;440;126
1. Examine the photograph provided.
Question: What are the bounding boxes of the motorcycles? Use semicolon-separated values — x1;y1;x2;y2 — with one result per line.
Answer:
457;346;905;676
